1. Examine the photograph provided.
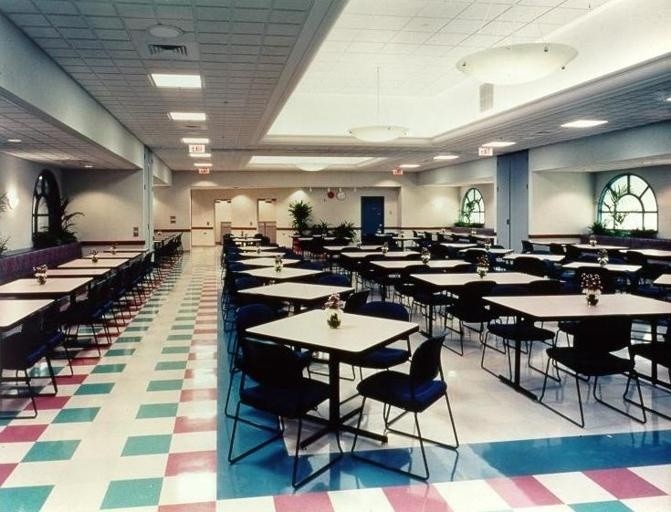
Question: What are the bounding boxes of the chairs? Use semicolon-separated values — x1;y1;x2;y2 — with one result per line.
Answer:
0;233;183;419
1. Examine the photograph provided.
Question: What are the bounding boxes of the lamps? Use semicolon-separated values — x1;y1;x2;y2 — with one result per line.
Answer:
298;157;327;172
349;67;408;143
457;1;578;87
